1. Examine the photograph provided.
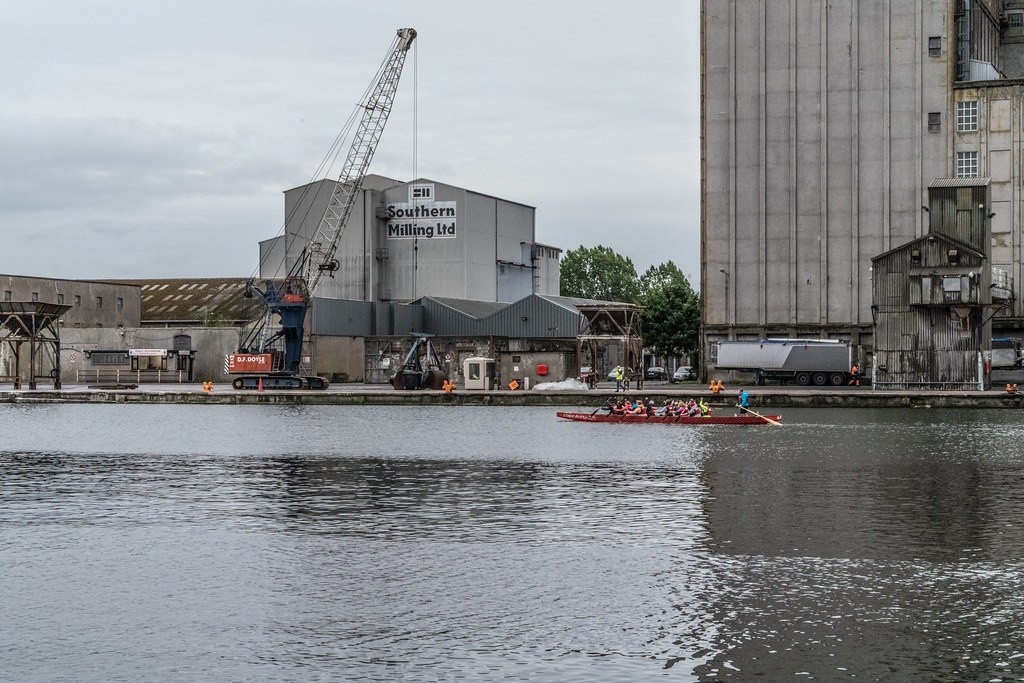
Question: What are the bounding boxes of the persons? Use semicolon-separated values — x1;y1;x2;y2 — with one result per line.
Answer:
614;366;626;392
738;388;749;417
600;397;712;418
847;364;859;387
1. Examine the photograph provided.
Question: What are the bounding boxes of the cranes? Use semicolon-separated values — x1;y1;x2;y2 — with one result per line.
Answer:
223;28;420;389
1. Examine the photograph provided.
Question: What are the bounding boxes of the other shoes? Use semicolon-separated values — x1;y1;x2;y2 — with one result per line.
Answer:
623;387;626;391
615;391;620;392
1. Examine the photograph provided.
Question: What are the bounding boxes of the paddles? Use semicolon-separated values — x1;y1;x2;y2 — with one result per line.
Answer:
700;397;703;417
618;410;627;425
644;406;661;421
605;409;612;421
661;412;670;422
734;402;785;427
590;395;613;418
675;401;691;422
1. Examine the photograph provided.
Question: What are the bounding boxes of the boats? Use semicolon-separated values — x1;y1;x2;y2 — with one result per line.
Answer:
557;412;782;424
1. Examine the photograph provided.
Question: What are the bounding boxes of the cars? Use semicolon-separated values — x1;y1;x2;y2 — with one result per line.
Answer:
581;366;600;383
673;366;696;382
646;367;668;381
607;366;633;382
